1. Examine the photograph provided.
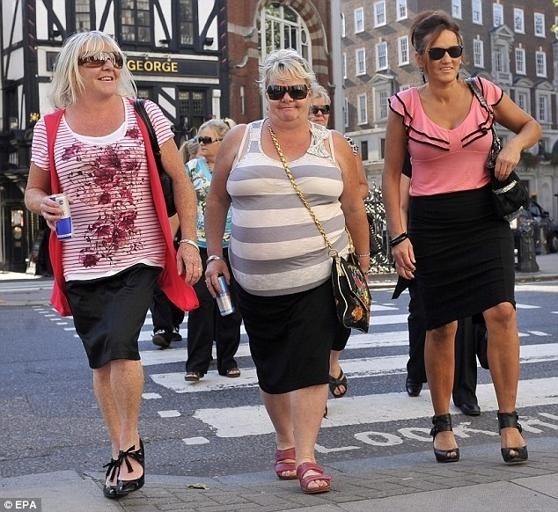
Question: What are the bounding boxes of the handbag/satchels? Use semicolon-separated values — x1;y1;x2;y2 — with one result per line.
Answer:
485;138;531;224
134;98;177;218
330;252;373;335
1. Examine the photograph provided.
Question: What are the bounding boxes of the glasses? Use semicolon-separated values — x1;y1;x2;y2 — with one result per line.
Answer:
78;50;124;70
428;45;464;60
199;136;223;145
266;84;310;100
311;105;332;114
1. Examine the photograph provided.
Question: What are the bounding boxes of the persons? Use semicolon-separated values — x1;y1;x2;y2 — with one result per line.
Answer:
179;136;200;164
24;30;204;498
307;82;369;418
381;10;542;462
185;117;242;381
205;48;371;493
391;144;489;416
150;137;185;347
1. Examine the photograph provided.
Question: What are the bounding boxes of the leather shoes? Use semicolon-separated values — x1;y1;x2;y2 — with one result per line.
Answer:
116;437;146;496
454;388;482;417
102;444;129;502
405;367;424;397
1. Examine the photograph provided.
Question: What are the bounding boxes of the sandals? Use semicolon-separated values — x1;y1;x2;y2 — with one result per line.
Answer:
184;367;204;383
274;444;298;480
296;460;332;494
220;365;241;378
329;365;349;400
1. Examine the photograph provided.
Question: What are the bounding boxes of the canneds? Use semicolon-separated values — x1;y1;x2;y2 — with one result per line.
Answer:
215;276;235;317
49;196;74;240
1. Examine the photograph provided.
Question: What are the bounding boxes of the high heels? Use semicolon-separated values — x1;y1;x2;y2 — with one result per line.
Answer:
430;413;460;464
497;408;528;463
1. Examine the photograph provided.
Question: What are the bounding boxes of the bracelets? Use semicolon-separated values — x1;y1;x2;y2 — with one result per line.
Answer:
180;239;200;252
354;253;370;257
206;255;224;264
389;232;407;247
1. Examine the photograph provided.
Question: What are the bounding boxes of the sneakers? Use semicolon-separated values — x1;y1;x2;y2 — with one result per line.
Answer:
151;326;172;349
167;323;183;343
